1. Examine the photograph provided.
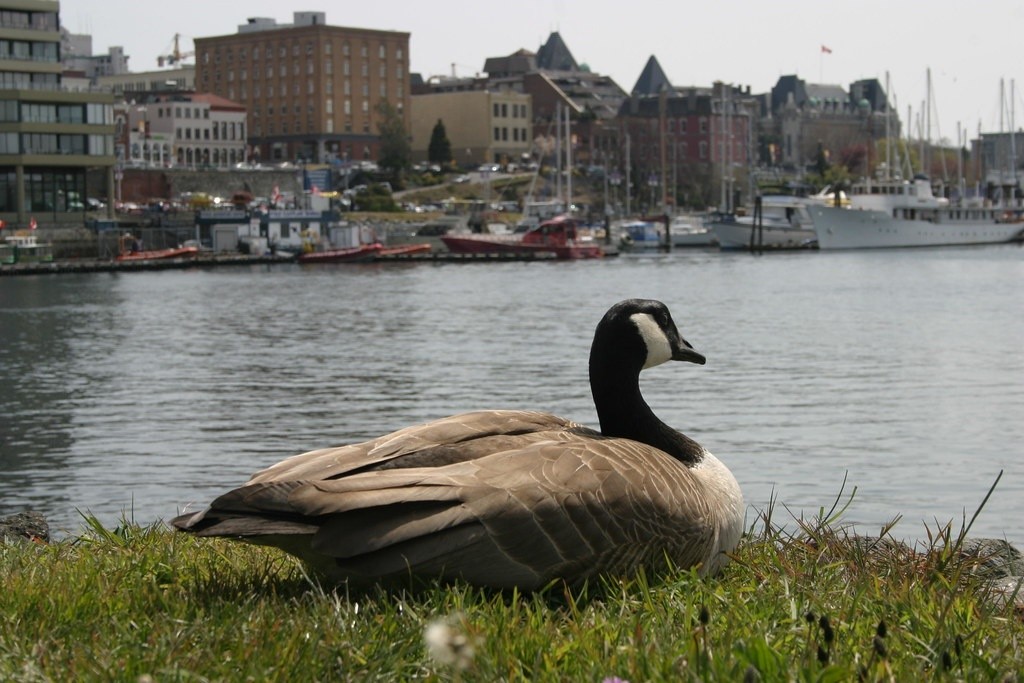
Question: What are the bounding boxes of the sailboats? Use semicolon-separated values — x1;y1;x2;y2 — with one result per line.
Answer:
444;65;1023;258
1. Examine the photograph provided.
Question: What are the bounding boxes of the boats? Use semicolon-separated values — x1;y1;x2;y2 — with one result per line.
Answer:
296;242;432;265
115;244;196;261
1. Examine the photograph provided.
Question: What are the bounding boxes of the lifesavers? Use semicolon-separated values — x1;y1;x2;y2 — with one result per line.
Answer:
618;232;635;247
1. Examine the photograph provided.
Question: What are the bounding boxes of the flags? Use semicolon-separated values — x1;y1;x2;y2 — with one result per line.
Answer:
29;218;38;231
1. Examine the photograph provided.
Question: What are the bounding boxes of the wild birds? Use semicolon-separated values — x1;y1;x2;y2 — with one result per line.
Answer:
169;297;748;608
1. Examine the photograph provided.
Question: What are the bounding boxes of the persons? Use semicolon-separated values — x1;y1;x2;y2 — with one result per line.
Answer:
301;225;317;253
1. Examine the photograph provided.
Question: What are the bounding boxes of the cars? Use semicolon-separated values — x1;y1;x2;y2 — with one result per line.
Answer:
84;192;294;212
234;157;379;173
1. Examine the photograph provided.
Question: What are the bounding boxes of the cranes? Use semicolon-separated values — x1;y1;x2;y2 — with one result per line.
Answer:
156;33;195;69
